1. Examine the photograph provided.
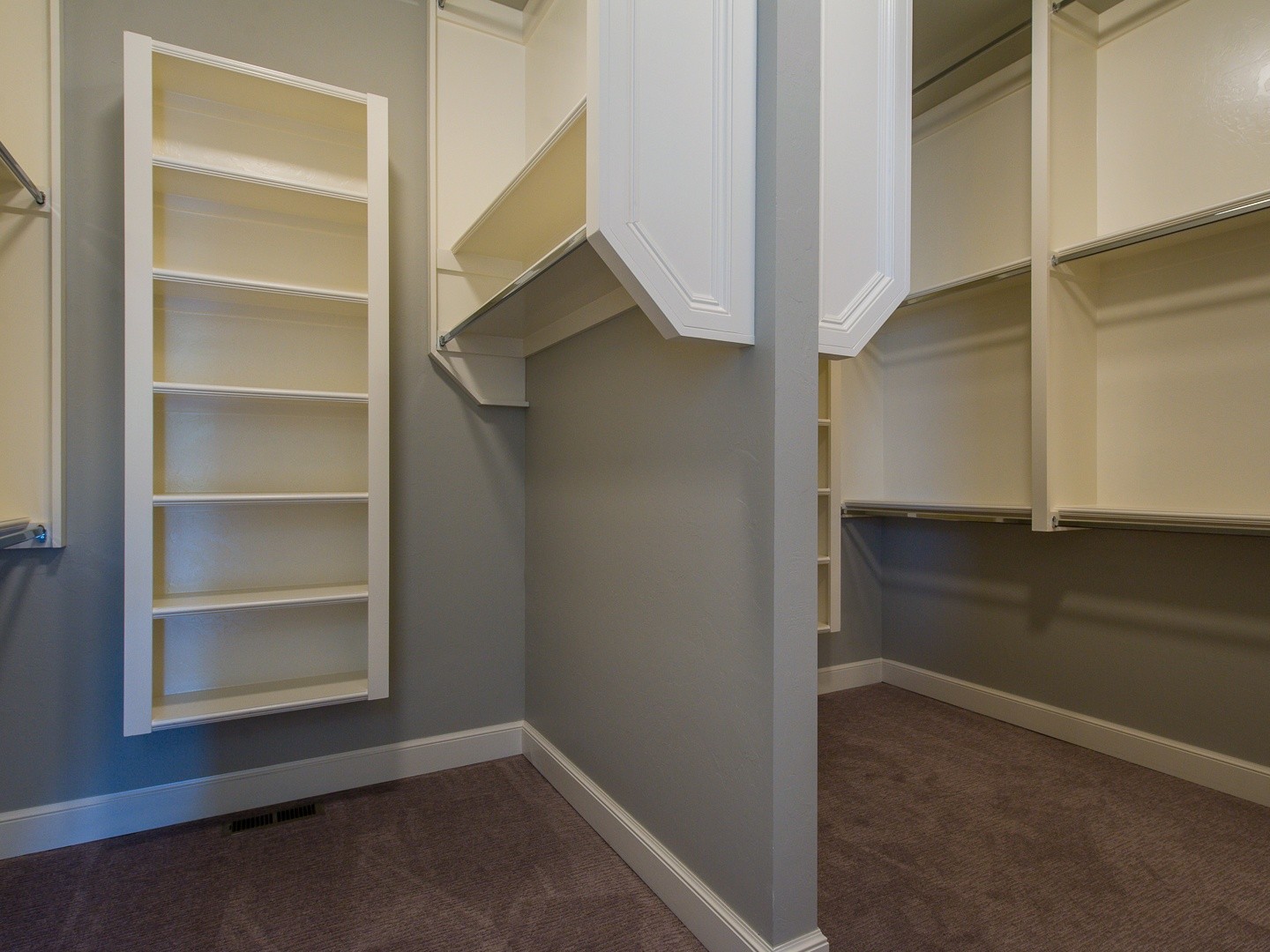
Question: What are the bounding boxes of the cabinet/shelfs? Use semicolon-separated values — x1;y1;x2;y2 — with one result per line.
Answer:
124;31;390;739
817;0;1270;634
428;0;756;406
0;1;70;550
